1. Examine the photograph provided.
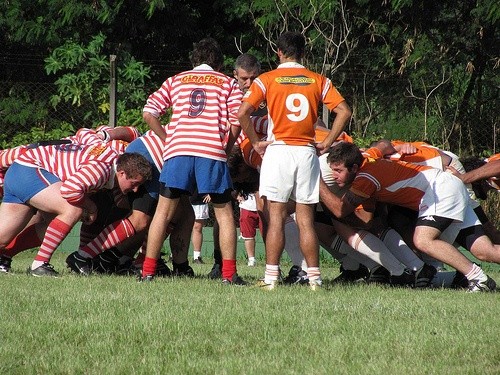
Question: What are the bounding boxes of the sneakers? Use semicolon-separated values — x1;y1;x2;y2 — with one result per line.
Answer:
64;250;222;284
329;260;497;297
280;265;311;288
26;261;63;278
221;275;255;289
249;278;280;292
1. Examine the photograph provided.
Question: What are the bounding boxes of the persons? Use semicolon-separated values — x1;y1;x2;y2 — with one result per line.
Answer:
237;33;352;290
232;54;268;116
0;116;500;296
142;39;245;286
